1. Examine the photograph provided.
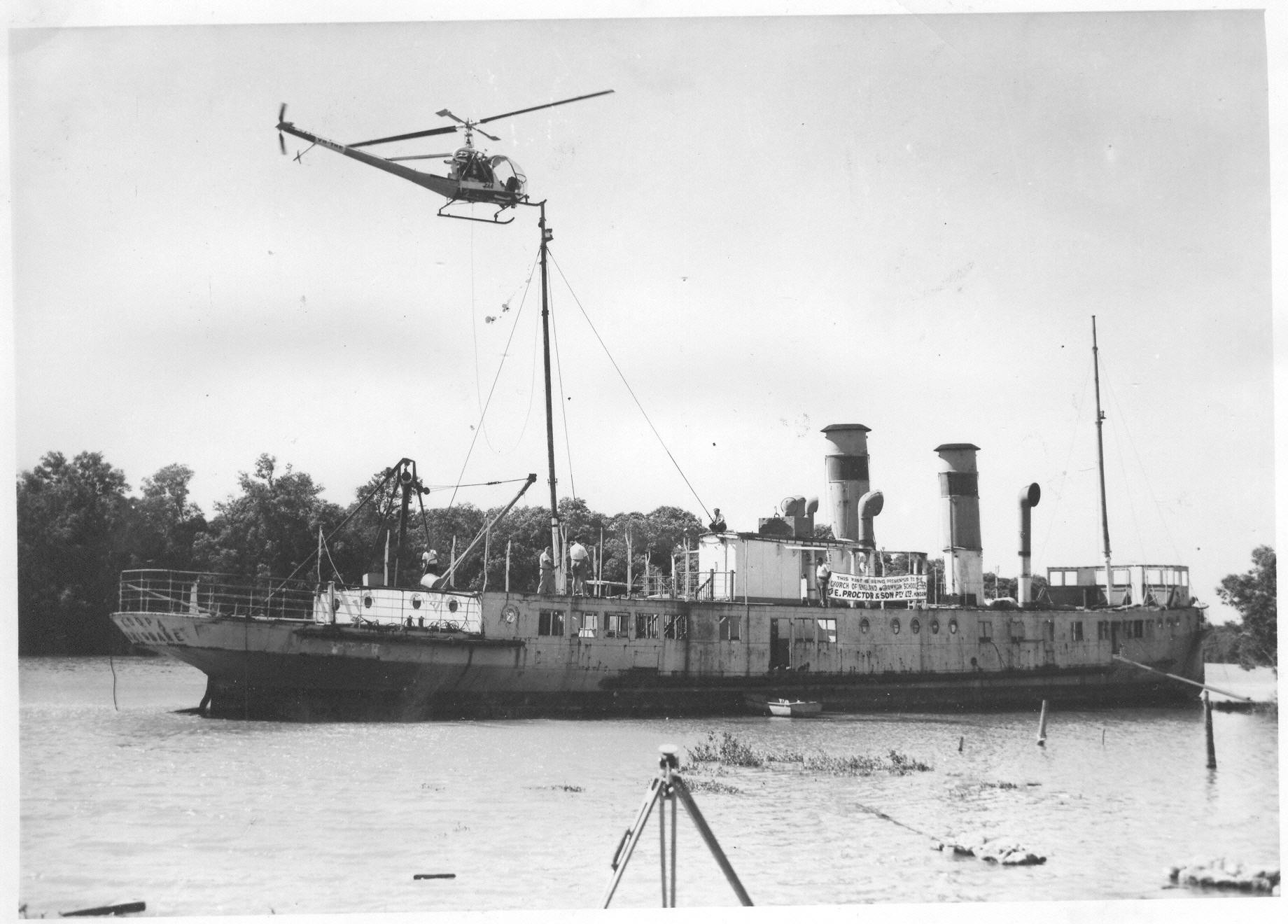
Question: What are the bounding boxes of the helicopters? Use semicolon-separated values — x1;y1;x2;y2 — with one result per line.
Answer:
277;88;615;225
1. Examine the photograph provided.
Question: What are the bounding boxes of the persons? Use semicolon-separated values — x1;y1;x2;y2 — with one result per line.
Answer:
568;536;590;598
815;557;832;607
420;543;438;576
708;508;727;534
536;546;558;592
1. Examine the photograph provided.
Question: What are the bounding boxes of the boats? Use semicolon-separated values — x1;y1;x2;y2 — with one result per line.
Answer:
103;204;1208;723
742;691;822;717
